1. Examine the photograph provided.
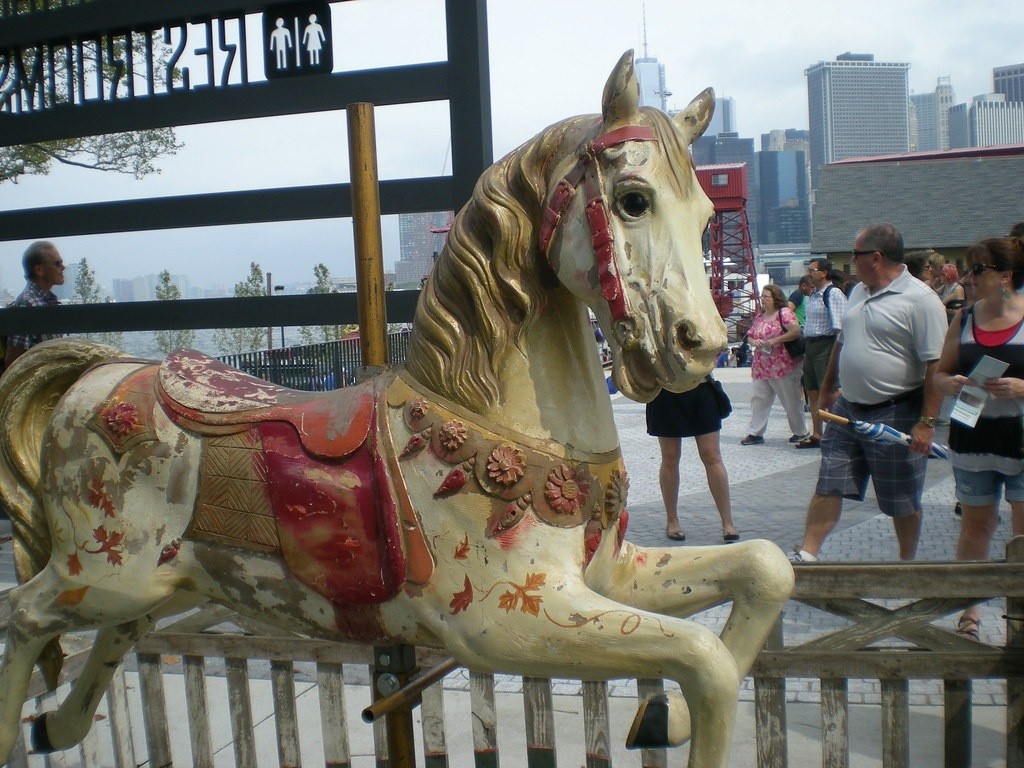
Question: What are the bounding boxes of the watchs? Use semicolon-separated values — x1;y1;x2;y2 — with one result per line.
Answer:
920;416;936;429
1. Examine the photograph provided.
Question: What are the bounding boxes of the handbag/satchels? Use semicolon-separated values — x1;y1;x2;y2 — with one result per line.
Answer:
779;307;806;357
946;285;967;309
704;373;732;419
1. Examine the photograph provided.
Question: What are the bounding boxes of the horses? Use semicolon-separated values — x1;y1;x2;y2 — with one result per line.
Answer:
0;48;799;766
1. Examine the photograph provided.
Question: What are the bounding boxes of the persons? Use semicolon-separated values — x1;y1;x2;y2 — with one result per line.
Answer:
718;222;1024;640
646;374;741;542
242;362;357;391
5;241;65;368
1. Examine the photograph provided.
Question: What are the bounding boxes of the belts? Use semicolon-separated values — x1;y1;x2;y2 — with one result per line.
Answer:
851;386;924;409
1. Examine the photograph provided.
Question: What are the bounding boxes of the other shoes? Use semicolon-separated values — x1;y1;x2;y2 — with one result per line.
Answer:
666;525;685;539
724;534;740;540
789;431;813;442
795;435;820;448
785;551;802;563
954;502;1002;525
956;612;980;642
935;416;951;425
740;435;765;445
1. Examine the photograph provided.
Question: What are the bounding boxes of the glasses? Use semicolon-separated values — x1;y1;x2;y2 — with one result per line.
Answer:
39;258;65;269
760;294;772;300
807;267;823;272
967;262;996;275
850;248;884;262
924;264;933;271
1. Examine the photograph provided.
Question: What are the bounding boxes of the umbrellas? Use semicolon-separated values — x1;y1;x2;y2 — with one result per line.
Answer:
816;409;949;460
